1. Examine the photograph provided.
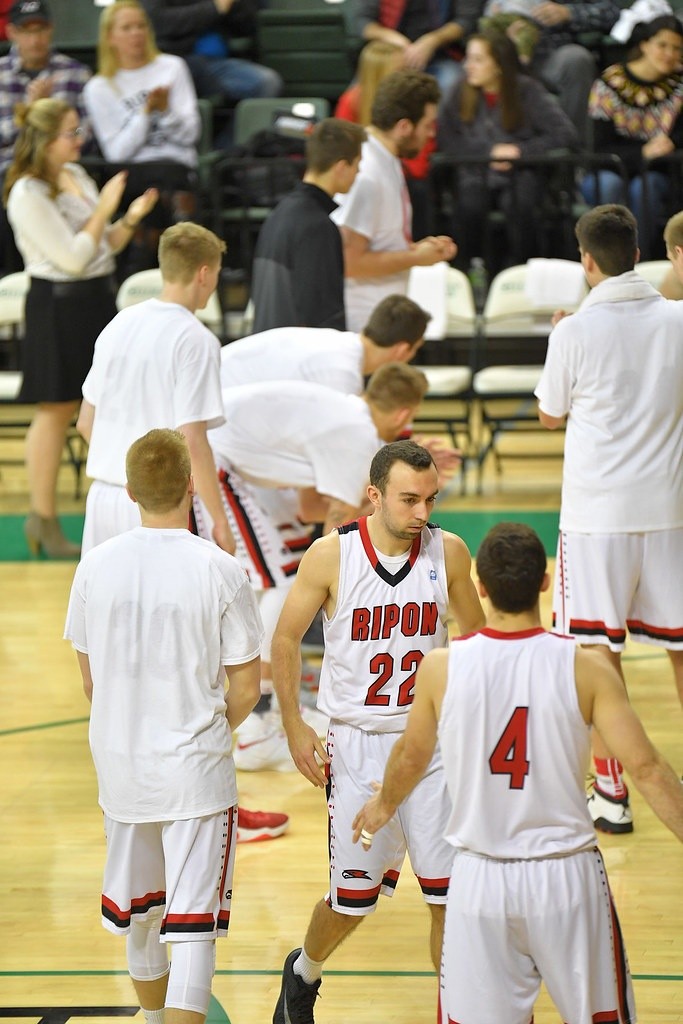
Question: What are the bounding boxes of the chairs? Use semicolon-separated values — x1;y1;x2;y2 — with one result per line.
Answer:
406;6;683;242
0;259;683;501
2;1;349;214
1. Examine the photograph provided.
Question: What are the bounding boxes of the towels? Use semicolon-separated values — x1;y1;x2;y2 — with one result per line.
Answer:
574;268;662;312
526;256;586;314
407;260;449;341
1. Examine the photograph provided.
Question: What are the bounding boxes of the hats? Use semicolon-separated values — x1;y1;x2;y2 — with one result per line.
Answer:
7;0;49;24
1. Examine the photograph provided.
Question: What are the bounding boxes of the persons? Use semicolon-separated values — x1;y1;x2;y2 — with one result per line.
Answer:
436;30;583;264
334;37;434;243
250;118;364;332
64;428;264;1024
194;296;459;771
347;0;486;108
659;211;683;301
143;0;282;146
352;524;682;1024
77;221;289;846
0;0;93;177
272;443;491;1023
1;98;156;559
83;0;201;191
328;71;457;332
535;205;682;834
486;0;620;124
583;15;683;240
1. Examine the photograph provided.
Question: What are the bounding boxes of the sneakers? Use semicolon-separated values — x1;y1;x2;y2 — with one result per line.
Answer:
238;807;290;844
585;782;631;834
271;949;322;1024
234;727;328;773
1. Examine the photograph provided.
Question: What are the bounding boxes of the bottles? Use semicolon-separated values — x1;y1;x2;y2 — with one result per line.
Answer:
467;257;486;309
274;115;317;140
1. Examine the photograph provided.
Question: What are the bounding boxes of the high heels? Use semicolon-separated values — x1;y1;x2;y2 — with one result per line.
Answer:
26;513;81;558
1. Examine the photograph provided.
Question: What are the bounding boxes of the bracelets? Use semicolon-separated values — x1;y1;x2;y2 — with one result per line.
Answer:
120;218;135;230
